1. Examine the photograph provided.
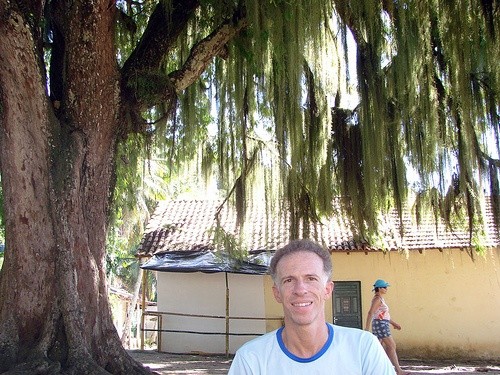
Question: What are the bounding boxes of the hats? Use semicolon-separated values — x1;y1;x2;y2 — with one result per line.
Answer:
372;280;389;292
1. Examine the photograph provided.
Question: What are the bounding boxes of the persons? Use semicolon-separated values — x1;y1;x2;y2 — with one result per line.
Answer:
226;239;398;375
364;280;411;375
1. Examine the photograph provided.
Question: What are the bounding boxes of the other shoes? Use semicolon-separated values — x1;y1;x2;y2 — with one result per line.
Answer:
402;371;411;375
